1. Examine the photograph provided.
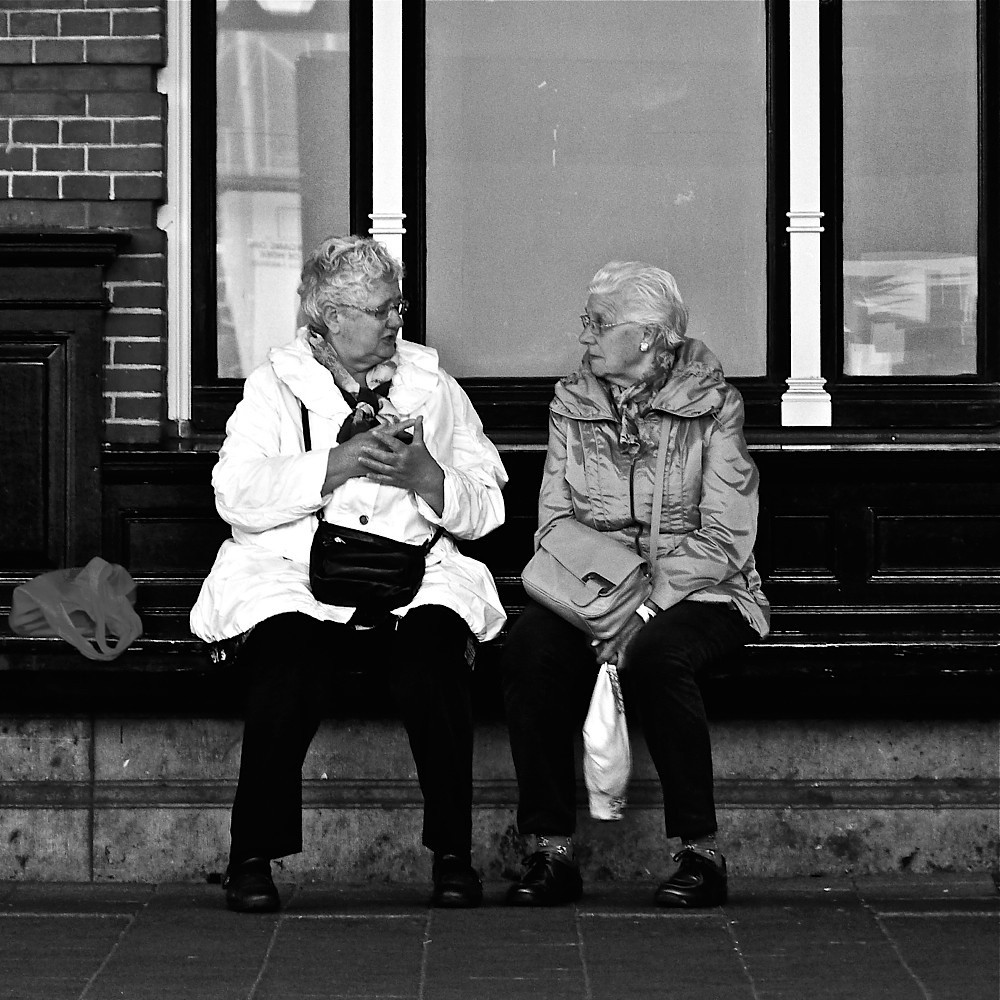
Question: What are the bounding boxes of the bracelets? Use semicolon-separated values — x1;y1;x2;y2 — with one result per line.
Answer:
635;602;657;625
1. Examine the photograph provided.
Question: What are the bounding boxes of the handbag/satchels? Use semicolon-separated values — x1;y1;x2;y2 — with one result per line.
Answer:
301;402;444;611
8;555;145;661
581;661;631;821
522;414;671;640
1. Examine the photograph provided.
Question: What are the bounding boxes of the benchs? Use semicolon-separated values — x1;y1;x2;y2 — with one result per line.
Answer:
0;442;1000;672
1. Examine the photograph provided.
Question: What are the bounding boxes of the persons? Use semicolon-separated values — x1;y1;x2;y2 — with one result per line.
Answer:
504;259;771;910
188;232;509;914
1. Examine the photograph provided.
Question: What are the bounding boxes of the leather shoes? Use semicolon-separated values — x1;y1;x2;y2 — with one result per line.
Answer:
433;856;485;907
654;848;730;907
222;859;281;907
509;850;584;906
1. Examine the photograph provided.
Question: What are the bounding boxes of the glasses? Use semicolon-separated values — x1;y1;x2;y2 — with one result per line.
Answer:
579;313;652;335
336;300;411;320
